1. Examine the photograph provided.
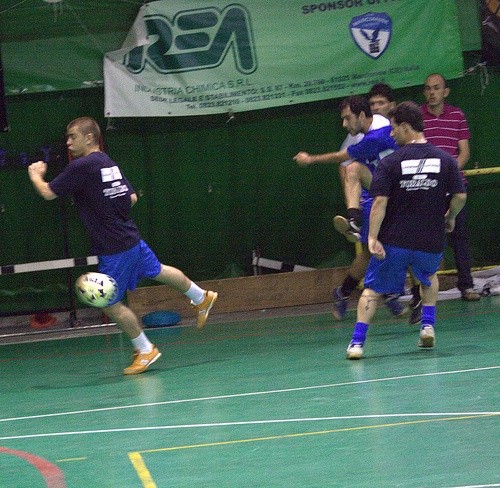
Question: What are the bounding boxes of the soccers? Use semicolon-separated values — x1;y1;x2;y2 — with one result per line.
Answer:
76;272;119;308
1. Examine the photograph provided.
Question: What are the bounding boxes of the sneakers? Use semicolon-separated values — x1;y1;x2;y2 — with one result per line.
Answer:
410;302;424;324
190;290;218;329
419;326;435;349
122;346;162;374
386;295;409;317
461;288;481;301
329;285;349;320
333;215;362;243
346;342;364;359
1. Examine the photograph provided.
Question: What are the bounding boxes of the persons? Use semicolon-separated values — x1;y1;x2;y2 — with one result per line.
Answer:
27;117;217;376
293;72;481;360
